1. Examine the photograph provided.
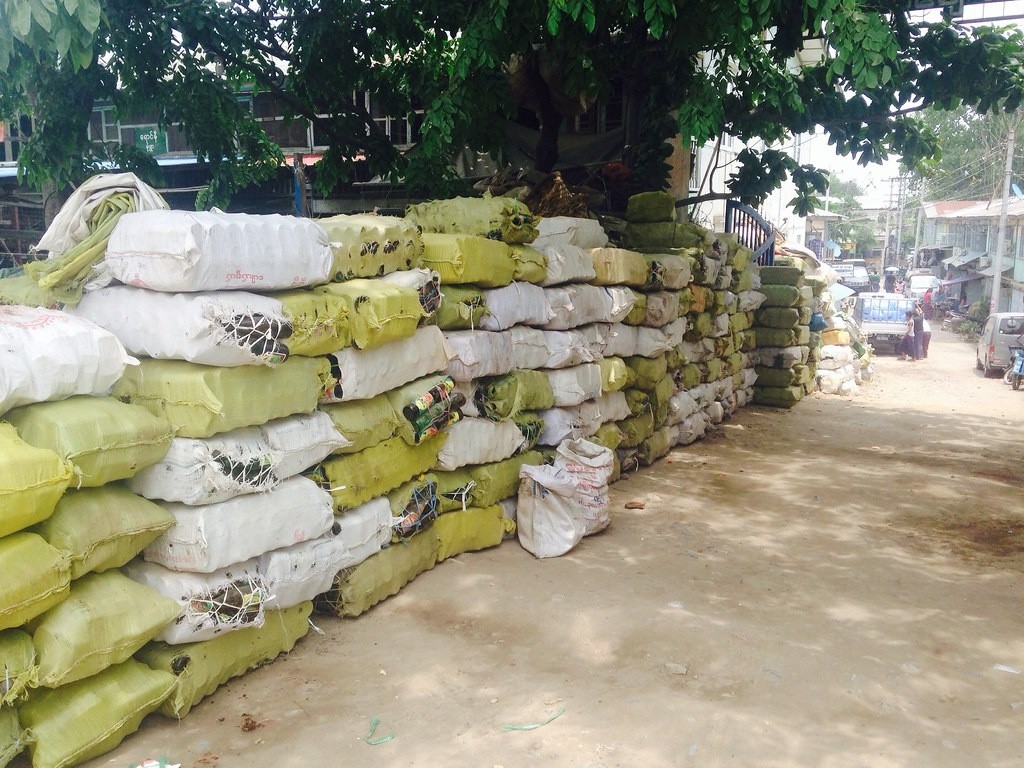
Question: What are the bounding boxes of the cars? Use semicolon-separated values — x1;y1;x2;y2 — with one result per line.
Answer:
975;312;1024;377
905;276;940;304
851;291;932;355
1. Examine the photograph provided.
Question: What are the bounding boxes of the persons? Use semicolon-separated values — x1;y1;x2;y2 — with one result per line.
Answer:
925;288;934;320
871;271;896;293
898;300;931;362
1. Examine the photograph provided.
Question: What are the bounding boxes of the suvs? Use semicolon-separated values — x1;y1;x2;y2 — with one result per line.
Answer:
837;265;871;293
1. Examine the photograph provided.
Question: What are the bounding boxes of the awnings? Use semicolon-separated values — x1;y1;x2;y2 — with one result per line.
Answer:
806;208;849;223
938;251;1013;286
918;244;953;253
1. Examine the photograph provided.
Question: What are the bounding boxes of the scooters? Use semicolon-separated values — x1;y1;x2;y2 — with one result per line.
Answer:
1001;333;1024;390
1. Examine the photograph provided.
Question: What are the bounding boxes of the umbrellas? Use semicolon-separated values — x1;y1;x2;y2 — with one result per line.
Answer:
886;267;899;274
828;283;856;302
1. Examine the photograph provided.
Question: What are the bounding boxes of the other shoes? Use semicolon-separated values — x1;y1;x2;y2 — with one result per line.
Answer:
898;356;905;361
908;359;915;362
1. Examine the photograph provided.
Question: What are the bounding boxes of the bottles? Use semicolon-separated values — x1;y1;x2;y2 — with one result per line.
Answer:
328;355;343;399
189;579;262;624
647;261;666;284
304;468;342;535
488;215;533;242
512;423;541;455
351;337;362;350
396;483;440;537
219;452;277;485
220;314;293;363
863;300;912;322
403;375;466;442
173;657;190;678
312;584;342;616
338;226;440;313
446;484;471;502
